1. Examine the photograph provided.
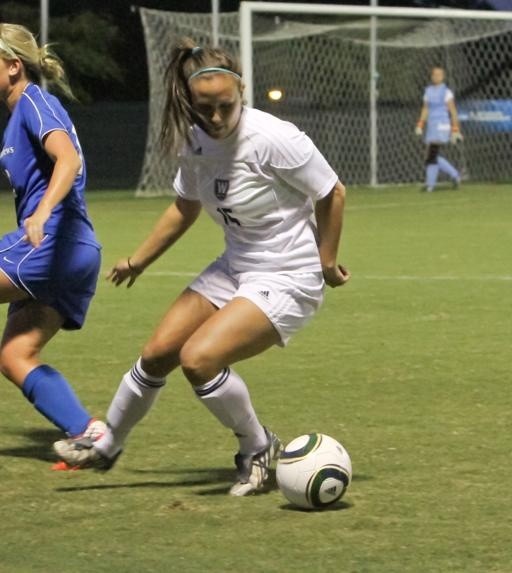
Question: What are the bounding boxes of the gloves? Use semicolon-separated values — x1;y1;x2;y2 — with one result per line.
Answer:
450;128;463;145
414;126;422;136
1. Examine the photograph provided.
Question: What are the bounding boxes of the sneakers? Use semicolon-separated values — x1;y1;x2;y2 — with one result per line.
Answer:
229;424;285;497
51;417;122;472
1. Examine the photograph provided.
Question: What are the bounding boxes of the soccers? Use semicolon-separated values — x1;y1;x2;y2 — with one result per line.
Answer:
276;433;352;509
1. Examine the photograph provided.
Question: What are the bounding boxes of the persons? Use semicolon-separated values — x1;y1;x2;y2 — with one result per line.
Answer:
1;21;103;474
414;66;464;193
48;40;354;496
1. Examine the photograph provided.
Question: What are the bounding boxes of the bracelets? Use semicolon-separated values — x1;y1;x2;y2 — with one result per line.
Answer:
127;255;140;273
